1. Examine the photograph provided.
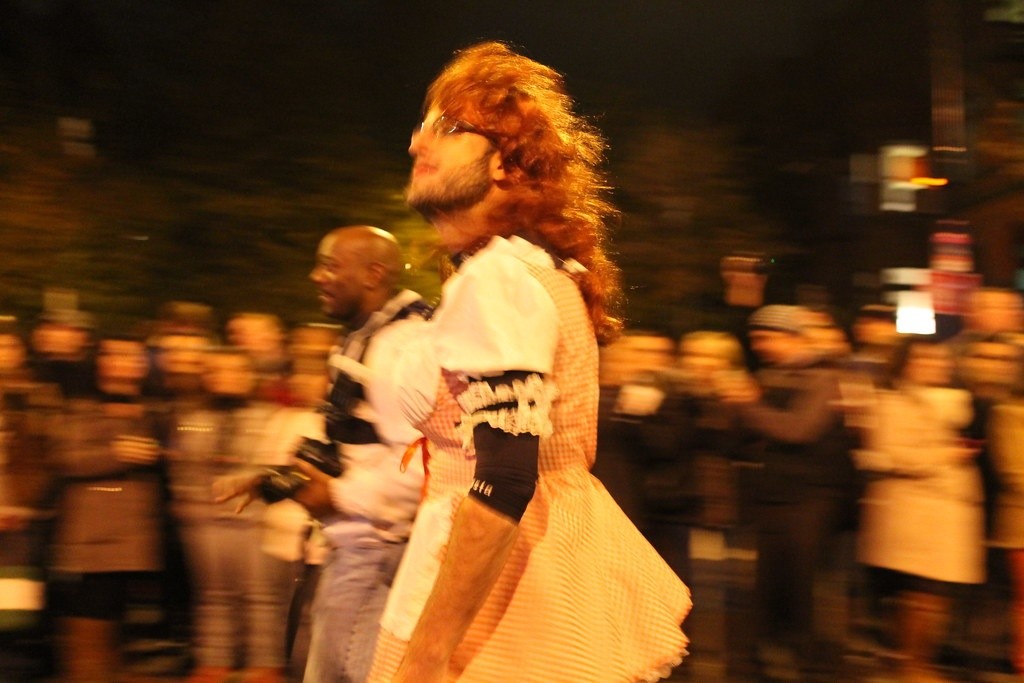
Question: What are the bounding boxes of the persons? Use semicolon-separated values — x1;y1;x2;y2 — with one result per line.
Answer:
0;219;1024;683
213;41;692;683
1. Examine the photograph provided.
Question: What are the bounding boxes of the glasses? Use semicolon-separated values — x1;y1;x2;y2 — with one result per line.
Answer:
409;117;497;145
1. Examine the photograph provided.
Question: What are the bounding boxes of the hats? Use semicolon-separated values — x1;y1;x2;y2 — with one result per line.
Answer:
750;303;799;333
